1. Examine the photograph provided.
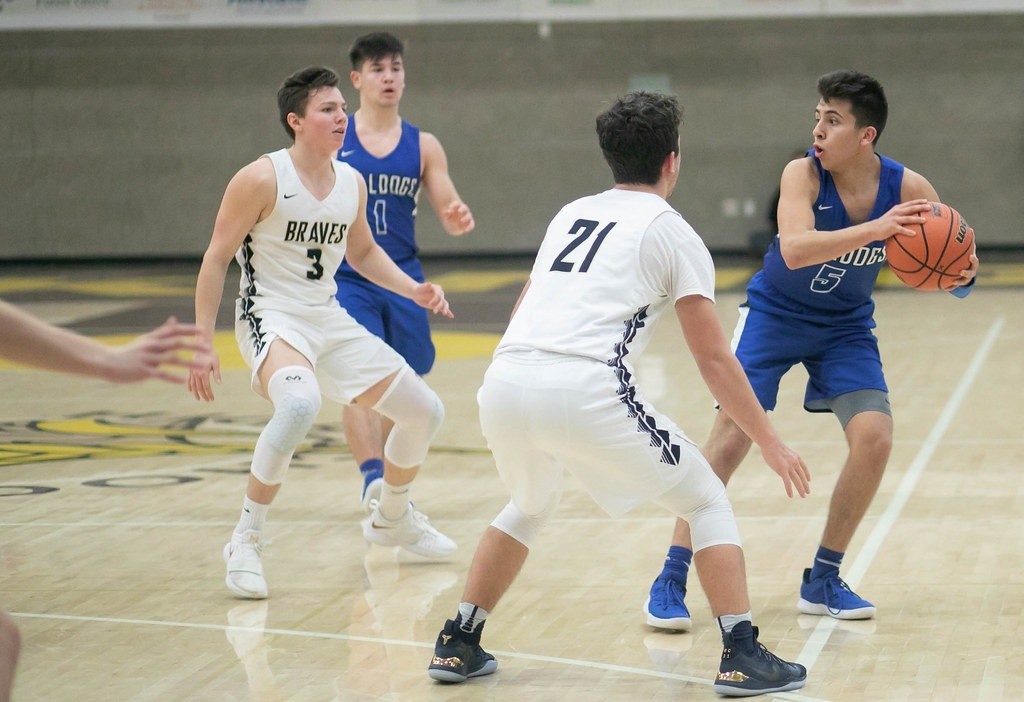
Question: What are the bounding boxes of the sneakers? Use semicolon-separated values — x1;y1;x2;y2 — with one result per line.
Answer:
798;568;876;620
361;469;384;515
362;498;457;558
428;619;498;682
713;626;808;695
223;530;268;598
643;570;692;630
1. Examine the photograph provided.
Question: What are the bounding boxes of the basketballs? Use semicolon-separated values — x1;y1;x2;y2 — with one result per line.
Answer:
886;200;976;292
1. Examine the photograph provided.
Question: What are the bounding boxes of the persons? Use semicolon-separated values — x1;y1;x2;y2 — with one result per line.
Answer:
642;71;980;630
332;32;475;518
428;89;811;698
187;65;460;600
0;298;208;700
